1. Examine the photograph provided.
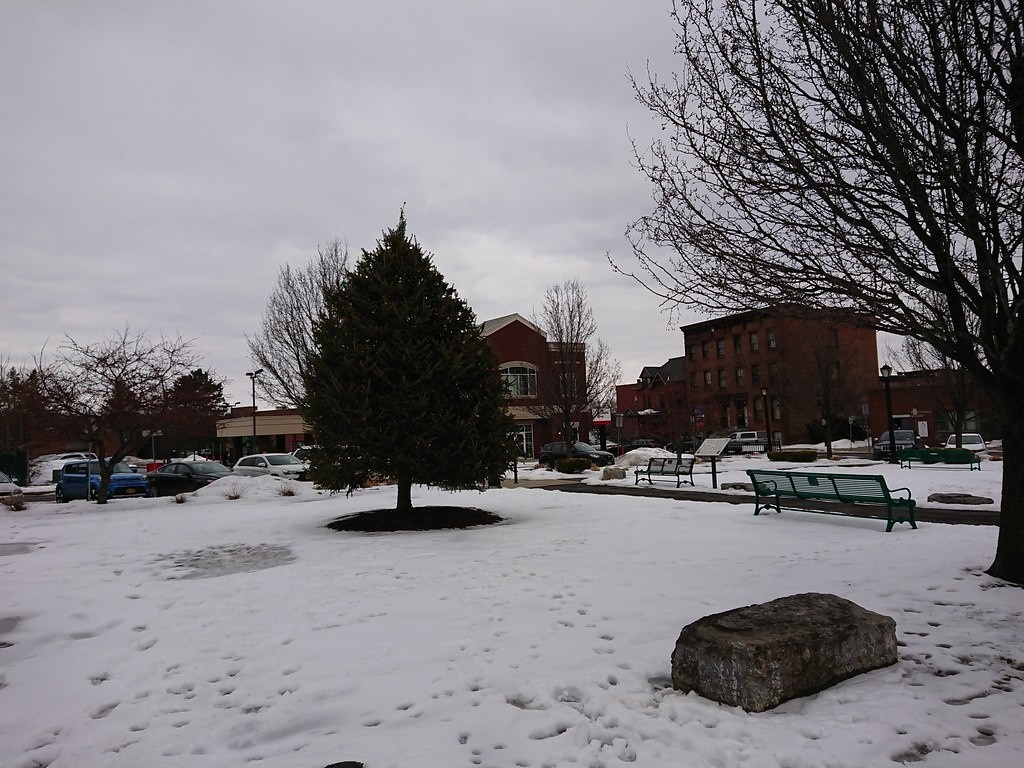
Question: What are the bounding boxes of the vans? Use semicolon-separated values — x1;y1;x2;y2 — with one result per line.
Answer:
725;430;773;454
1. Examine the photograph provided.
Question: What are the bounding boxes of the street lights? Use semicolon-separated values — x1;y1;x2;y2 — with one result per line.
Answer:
880;364;903;463
143;429;163;463
245;369;262;449
760;385;773;451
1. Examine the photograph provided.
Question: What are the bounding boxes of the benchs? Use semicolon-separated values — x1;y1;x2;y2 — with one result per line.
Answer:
746;469;917;532
634;458;696;488
898;448;981;471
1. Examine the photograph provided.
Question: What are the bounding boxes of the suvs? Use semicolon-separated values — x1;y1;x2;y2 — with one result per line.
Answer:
539;440;615;469
623;438;658;453
872;429;922;462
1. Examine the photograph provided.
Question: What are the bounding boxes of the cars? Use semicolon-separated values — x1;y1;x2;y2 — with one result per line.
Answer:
665;435;703;455
289;443;393;482
941;432;992;459
0;471;24;510
145;461;233;497
56;457;150;503
590;437;622;456
27;451;217;486
235;452;308;482
